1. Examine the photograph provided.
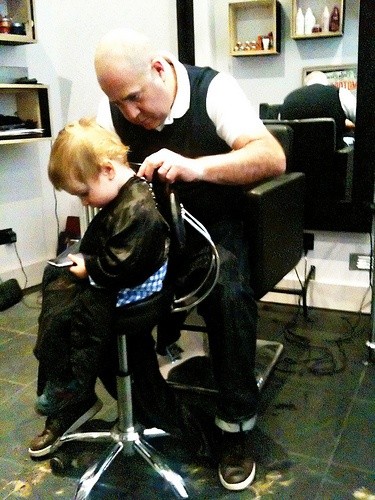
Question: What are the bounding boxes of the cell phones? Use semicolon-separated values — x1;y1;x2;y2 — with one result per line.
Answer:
47;258;77;267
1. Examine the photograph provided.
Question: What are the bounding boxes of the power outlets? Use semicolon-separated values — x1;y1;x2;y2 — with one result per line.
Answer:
0;228;12;245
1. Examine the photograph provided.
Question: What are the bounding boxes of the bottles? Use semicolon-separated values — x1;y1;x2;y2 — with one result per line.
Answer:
295;7;305;35
321;6;330;31
234;32;273;51
329;7;340;32
304;7;316;34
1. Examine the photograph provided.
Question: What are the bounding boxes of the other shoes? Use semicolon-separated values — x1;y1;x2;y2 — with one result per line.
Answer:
218;429;256;491
33;378;83;416
28;390;103;456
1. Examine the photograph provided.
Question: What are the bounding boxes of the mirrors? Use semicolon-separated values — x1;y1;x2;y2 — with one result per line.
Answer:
175;0;361;202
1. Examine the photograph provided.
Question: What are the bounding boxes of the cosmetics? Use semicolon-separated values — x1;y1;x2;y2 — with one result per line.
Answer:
234;31;273;51
295;5;339;35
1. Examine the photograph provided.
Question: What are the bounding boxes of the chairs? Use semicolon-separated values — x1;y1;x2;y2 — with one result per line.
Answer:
49;188;220;500
150;123;309;399
262;116;355;202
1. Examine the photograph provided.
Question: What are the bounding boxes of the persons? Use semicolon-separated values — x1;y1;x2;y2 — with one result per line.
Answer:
279;70;357;150
28;117;169;456
94;33;286;491
